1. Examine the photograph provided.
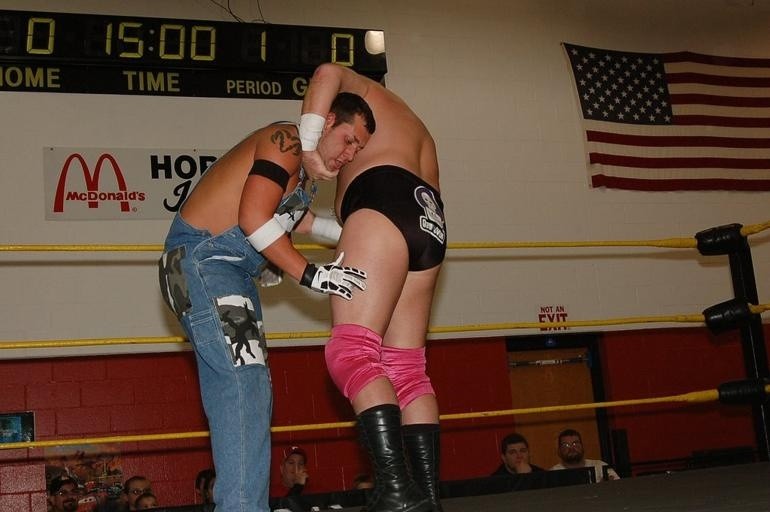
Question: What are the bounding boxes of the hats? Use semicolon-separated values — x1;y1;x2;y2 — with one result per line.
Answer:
50;472;78;494
281;444;308;465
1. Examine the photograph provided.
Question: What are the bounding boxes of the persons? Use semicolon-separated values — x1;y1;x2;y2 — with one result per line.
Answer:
157;92;380;510
48;472;81;511
491;434;544;476
193;470;216;511
549;429;621;484
353;476;375;489
121;475;151;510
134;492;157;510
280;446;311;511
296;65;442;510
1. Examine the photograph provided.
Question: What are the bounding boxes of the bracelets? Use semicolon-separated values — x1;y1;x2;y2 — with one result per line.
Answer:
313;214;345;247
300;112;326;153
245;219;287;253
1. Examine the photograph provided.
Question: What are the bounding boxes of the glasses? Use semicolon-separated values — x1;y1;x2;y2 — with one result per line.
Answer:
127;487;152;495
54;489;78;496
559;440;583;449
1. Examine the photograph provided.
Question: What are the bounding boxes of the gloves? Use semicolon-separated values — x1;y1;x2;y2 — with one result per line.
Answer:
299;252;367;300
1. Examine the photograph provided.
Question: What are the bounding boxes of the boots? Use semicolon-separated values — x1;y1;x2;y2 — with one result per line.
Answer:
398;417;445;512
352;402;436;512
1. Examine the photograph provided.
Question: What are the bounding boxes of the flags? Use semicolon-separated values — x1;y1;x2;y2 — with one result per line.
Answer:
562;42;770;199
